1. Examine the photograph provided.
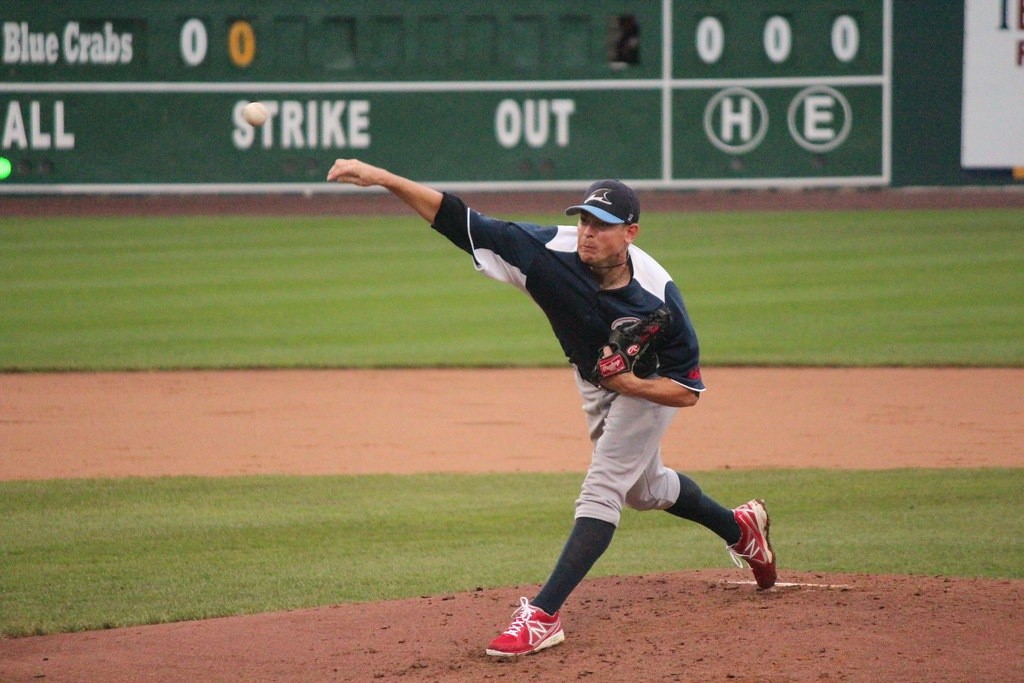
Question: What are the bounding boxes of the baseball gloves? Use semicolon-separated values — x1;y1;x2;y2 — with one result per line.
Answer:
594;305;673;377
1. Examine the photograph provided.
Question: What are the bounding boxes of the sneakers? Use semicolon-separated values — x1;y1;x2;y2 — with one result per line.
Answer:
726;499;777;589
487;597;565;656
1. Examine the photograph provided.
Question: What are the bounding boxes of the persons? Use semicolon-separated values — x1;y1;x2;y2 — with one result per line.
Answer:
326;158;776;656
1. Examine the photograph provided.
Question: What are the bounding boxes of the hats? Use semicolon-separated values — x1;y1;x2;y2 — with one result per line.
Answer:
566;179;641;224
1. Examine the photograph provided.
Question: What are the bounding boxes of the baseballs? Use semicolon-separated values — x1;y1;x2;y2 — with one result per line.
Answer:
242;100;269;126
